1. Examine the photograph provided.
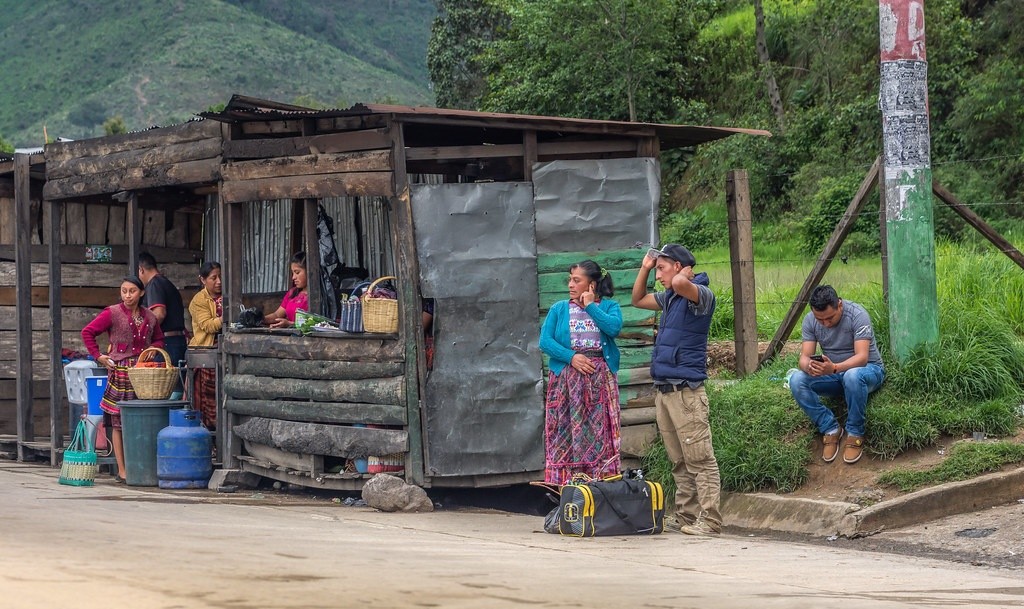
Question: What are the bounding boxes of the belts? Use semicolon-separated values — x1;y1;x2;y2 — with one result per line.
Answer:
659;380;704;394
576;351;603;357
162;330;186;337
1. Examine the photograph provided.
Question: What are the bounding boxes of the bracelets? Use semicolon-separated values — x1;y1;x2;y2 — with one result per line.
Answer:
833;363;837;373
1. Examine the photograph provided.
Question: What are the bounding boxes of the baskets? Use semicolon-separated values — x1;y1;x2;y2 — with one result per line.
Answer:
362;276;400;334
128;348;181;400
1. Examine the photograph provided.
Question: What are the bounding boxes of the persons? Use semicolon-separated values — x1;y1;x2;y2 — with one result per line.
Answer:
82;276;166;481
539;260;623;491
129;253;186;368
183;261;222;456
263;251;308;328
631;244;724;535
789;286;884;463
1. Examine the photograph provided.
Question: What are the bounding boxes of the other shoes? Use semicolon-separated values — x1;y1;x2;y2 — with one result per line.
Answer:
211;446;217;458
114;474;126;484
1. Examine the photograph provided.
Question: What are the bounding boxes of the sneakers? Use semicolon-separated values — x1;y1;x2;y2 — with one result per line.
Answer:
823;426;843;462
843;436;864;464
681;511;720;536
663;514;693;536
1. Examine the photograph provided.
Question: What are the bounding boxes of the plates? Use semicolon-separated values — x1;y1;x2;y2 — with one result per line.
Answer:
310;326;339;331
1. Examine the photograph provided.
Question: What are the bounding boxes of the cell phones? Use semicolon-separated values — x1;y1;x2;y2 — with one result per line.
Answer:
810;355;824;363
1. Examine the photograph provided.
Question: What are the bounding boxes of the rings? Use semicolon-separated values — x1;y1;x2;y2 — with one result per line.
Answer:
150;355;154;358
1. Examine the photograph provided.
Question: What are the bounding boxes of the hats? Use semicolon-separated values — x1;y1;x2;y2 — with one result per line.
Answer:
648;244;696;268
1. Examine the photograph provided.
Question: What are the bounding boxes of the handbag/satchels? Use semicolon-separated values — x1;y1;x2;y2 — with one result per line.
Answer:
240;302;267;327
58;419;97;486
529;454;620;534
559;475;665;537
339;282;379;332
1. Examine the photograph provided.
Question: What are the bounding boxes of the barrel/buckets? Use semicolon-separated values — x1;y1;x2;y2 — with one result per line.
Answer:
116;400;191;486
64;359;98;405
84;376;110;415
68;402;88;450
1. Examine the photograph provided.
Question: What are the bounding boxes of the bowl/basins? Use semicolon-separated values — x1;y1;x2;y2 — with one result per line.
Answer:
91;367;108;376
